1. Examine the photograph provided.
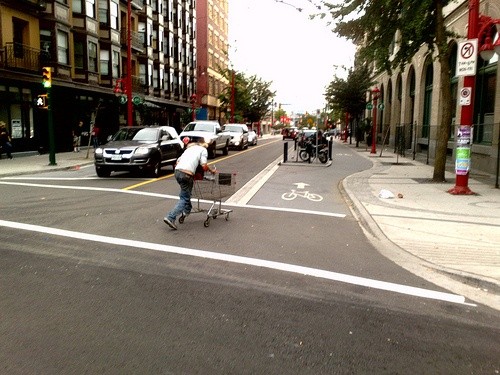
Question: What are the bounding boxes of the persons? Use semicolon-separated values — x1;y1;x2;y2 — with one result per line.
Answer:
164;142;216;230
313;128;323;144
0;120;13;159
72;120;99;152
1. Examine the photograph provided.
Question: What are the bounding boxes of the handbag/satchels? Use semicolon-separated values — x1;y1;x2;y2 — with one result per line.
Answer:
193;165;204;180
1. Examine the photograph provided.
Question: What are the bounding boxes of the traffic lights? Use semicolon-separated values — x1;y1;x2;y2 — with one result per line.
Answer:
41;65;53;90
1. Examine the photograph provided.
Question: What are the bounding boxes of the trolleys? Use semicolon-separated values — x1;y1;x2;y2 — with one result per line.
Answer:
179;166;238;227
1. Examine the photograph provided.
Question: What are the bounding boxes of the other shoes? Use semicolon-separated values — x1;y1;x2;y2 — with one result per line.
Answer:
183;211;190;217
164;217;179;230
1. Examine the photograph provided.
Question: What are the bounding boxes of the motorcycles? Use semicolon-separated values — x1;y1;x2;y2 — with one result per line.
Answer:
300;142;328;163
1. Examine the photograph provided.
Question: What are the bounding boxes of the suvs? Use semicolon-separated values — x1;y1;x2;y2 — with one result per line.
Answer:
93;125;184;178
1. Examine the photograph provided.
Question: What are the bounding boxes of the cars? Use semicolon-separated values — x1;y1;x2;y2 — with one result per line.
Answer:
246;130;258;145
221;123;248;150
281;127;295;140
298;127;318;141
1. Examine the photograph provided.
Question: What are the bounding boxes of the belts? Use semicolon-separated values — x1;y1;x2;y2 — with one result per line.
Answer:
179;171;193;177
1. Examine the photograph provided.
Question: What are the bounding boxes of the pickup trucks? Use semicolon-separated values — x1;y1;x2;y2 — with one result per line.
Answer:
180;120;231;155
